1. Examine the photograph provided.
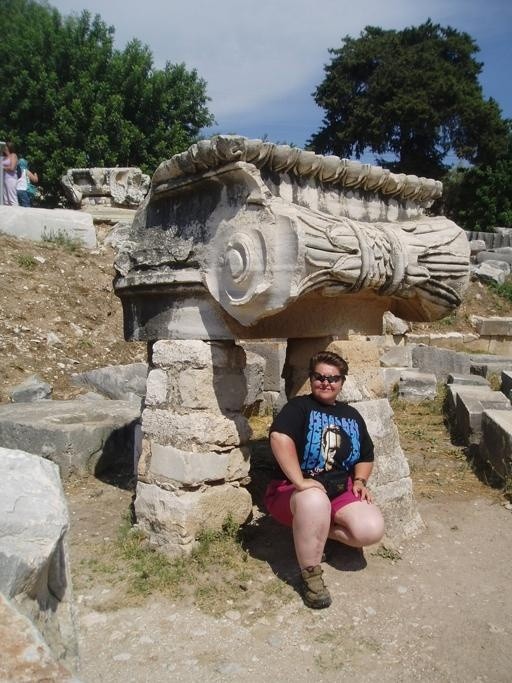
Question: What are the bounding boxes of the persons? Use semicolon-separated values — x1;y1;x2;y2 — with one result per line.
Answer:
0;142;19;207
263;350;385;608
18;157;39;208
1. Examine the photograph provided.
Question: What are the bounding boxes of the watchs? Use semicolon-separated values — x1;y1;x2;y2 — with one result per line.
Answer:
352;476;367;486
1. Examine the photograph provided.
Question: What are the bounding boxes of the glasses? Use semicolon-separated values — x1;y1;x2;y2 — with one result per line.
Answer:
311;371;342;383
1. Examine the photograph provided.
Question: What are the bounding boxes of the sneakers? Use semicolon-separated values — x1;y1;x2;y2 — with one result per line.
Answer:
300;566;332;609
